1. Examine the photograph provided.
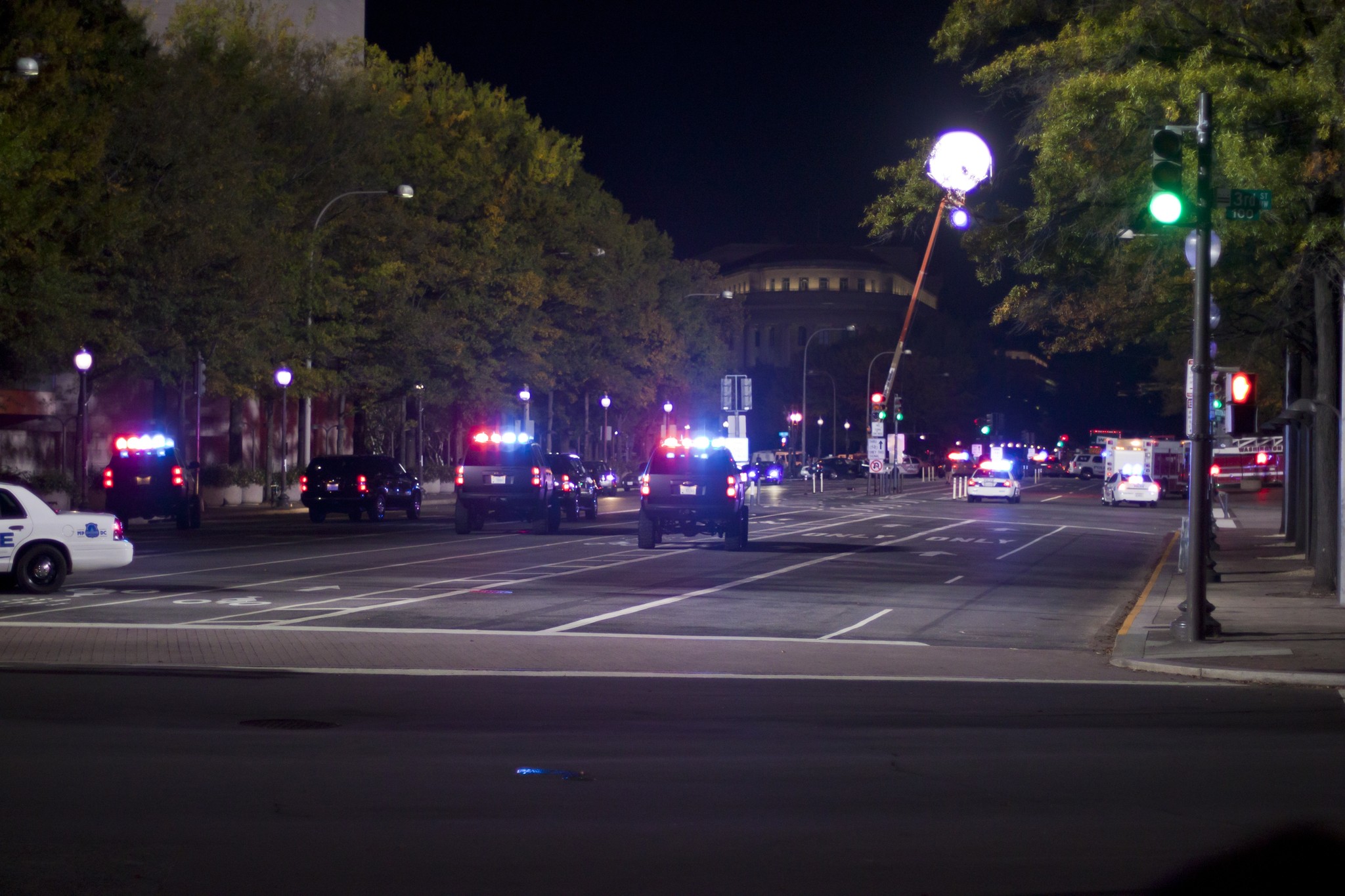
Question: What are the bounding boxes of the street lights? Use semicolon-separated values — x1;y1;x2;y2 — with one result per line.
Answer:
534;429;593;457
312;424;345;455
789;413;801;443
277;367;291;494
816;416;823;455
663;400;672;438
867;350;912;454
377;426;410;459
802;325;855;469
73;348;92;512
303;185;415;452
433;428;465;467
844;419;851;458
810;371;835;456
601;392;610;463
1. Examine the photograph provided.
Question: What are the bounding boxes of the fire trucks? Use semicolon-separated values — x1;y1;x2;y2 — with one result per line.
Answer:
1102;438;1285;498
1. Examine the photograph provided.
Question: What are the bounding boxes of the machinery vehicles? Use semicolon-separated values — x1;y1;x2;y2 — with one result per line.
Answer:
853;193;965;458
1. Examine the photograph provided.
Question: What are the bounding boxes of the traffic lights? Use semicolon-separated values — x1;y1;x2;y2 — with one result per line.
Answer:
1224;373;1258;435
192;361;206;395
1213;400;1223;410
1149;126;1184;226
1057;441;1063;448
893;398;903;423
974;414;993;434
870;393;886;422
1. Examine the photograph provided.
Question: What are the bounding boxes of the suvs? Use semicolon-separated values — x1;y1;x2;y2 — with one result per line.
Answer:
301;454;422;522
96;447;203;537
454;440;616;534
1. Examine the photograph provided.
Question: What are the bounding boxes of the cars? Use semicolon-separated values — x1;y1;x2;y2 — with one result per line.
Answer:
0;481;134;594
1100;473;1159;507
966;467;1021;503
949;450;1107;480
785;457;932;481
622;445;784;551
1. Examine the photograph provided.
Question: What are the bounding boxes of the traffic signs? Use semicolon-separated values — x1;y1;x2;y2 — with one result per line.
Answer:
971;444;982;455
867;438;886;461
